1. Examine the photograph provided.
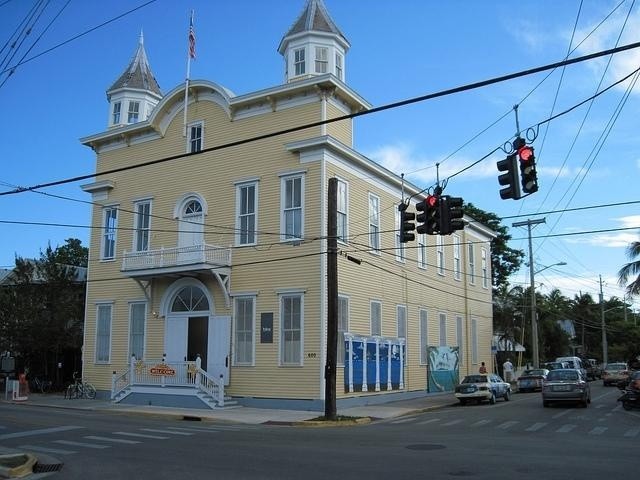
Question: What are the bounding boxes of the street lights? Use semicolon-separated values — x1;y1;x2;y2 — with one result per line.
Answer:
532;261;567;368
601;306;623;362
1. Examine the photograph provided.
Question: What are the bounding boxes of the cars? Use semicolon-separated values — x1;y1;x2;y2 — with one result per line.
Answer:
454;357;640;408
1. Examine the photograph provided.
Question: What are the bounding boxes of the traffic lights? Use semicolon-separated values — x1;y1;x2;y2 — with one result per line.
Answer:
398;194;465;243
497;146;539;200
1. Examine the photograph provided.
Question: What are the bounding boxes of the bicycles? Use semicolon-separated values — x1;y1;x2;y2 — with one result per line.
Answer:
30;375;50;393
66;372;96;399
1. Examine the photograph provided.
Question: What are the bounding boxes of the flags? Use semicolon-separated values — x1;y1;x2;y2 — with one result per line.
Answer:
189;9;196;58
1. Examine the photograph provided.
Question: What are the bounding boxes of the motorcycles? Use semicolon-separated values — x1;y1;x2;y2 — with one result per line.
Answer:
618;389;640;410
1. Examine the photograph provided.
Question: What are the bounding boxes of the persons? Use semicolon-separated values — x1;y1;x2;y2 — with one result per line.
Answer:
503;358;513;383
19;372;27;397
479;362;487;373
